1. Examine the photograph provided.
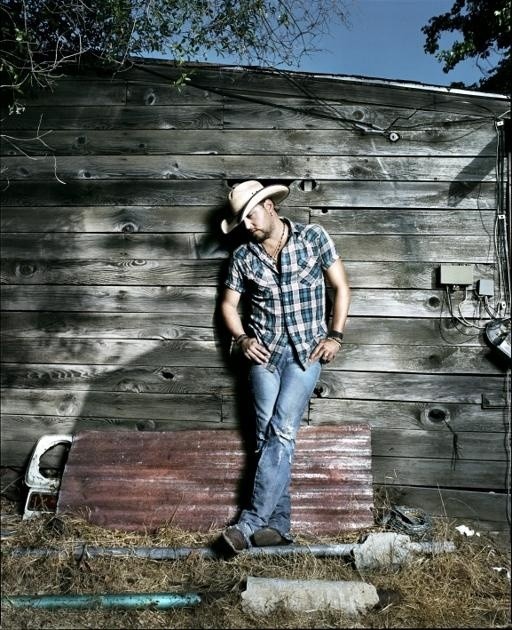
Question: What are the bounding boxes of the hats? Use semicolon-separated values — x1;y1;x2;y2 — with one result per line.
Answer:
221;180;290;235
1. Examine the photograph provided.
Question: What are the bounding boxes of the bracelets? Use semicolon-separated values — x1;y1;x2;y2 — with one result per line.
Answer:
328;330;343;342
236;334;248;345
328;338;342;347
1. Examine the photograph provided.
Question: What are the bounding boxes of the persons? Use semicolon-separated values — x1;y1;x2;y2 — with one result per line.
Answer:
221;179;350;553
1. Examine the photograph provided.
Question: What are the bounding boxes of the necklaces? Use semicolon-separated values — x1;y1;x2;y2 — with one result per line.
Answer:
270;222;286;257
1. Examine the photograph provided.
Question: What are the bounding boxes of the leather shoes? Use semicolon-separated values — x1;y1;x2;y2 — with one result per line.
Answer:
254;528;293;546
222;526;246;553
